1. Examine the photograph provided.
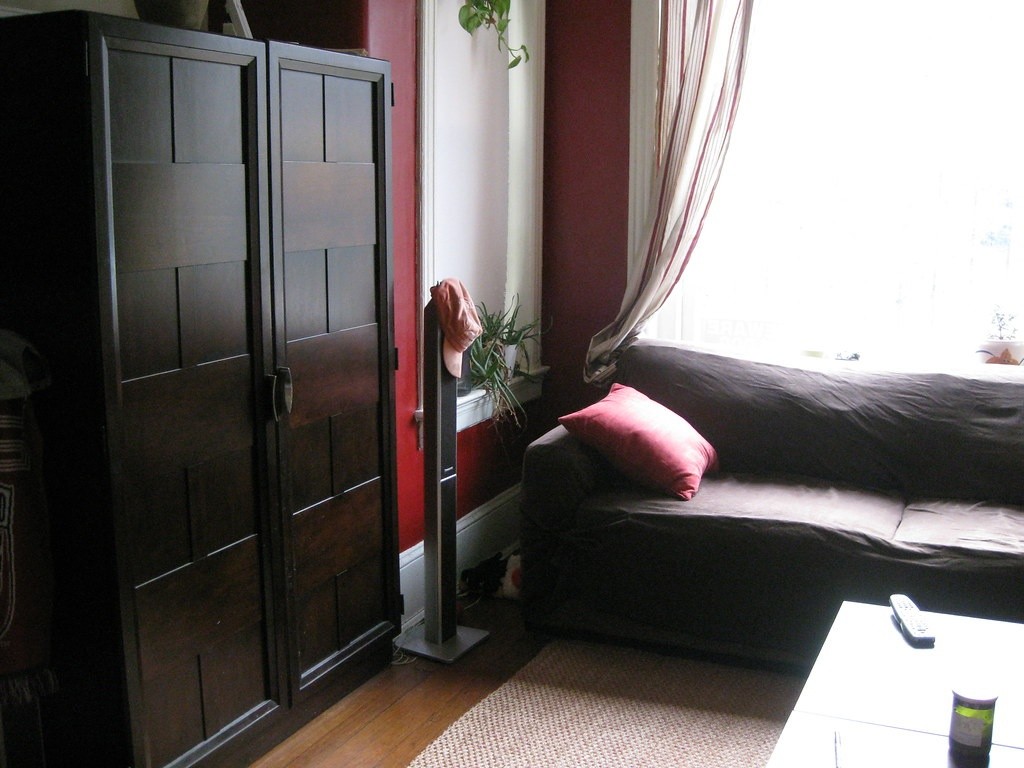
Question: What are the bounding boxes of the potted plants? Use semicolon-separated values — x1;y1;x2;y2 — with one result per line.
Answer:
974;303;1024;365
456;292;554;445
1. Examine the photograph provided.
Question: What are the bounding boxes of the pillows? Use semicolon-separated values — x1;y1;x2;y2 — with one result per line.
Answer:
558;383;719;501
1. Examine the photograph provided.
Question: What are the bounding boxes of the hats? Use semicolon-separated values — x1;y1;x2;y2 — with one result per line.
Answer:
431;278;483;378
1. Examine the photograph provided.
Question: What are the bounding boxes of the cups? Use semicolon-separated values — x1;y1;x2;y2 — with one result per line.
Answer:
134;0;208;30
950;690;997;747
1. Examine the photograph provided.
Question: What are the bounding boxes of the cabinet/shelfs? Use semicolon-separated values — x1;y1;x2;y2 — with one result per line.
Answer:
0;10;405;768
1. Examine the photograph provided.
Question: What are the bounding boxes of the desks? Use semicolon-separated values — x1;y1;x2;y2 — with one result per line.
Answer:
764;601;1023;768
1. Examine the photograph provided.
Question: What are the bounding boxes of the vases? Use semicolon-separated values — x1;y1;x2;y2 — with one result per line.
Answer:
133;0;209;30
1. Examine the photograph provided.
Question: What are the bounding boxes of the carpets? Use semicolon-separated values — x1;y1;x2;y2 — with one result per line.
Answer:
407;637;809;768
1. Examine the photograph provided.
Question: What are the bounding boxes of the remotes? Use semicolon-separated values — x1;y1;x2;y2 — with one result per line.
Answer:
889;594;935;644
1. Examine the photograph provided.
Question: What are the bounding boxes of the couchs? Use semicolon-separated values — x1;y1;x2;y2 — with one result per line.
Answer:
514;338;1024;666
